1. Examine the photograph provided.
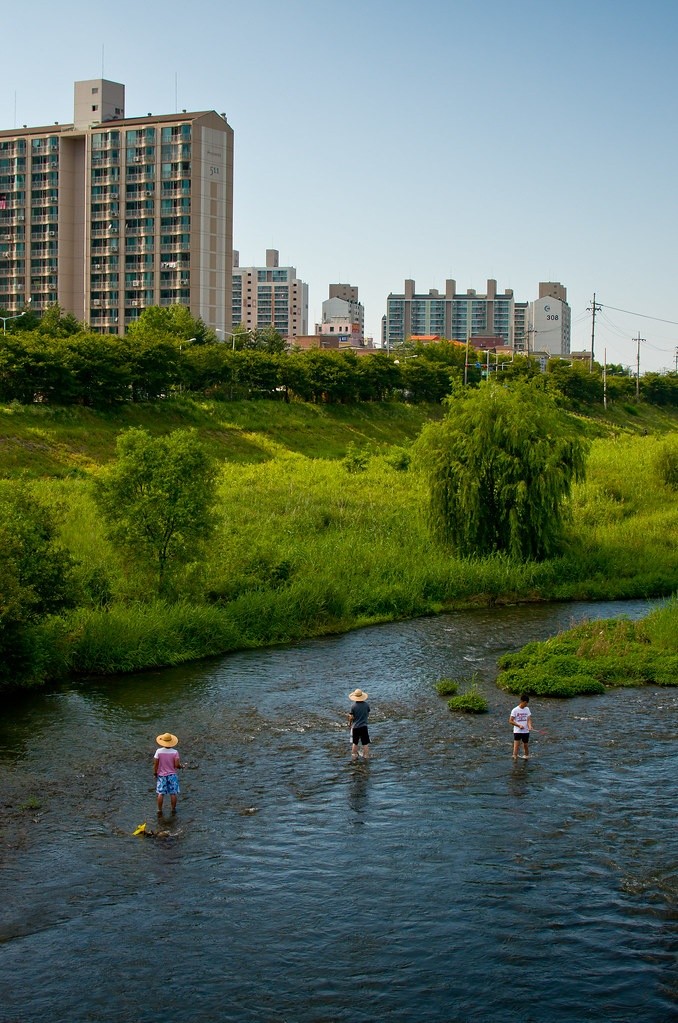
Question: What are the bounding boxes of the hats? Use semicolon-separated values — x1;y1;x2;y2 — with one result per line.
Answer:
349;689;368;701
156;733;178;747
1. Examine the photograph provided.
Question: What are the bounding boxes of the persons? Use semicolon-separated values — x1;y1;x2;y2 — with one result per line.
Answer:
348;689;371;758
509;695;533;757
152;733;185;818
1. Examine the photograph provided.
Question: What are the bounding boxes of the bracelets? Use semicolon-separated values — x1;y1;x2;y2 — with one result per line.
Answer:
153;772;157;775
